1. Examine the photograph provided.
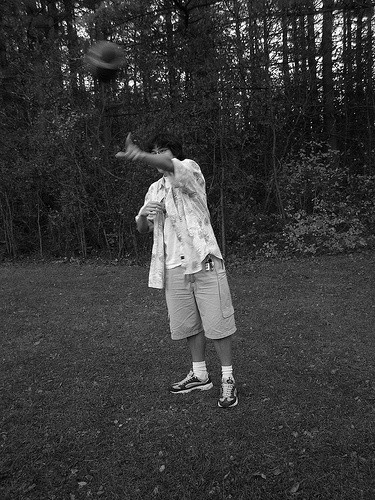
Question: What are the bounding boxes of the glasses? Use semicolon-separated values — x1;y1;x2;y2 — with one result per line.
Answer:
151;149;168;154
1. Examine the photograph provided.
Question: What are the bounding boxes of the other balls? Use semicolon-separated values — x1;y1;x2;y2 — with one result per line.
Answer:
86;40;123;80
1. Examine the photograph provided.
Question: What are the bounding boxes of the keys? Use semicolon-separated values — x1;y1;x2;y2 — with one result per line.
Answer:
204;257;215;272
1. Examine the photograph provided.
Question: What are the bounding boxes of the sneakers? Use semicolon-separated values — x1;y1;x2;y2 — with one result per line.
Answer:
218;380;239;407
169;371;213;393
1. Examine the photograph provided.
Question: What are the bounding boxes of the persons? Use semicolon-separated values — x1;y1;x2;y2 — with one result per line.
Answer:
116;131;239;408
